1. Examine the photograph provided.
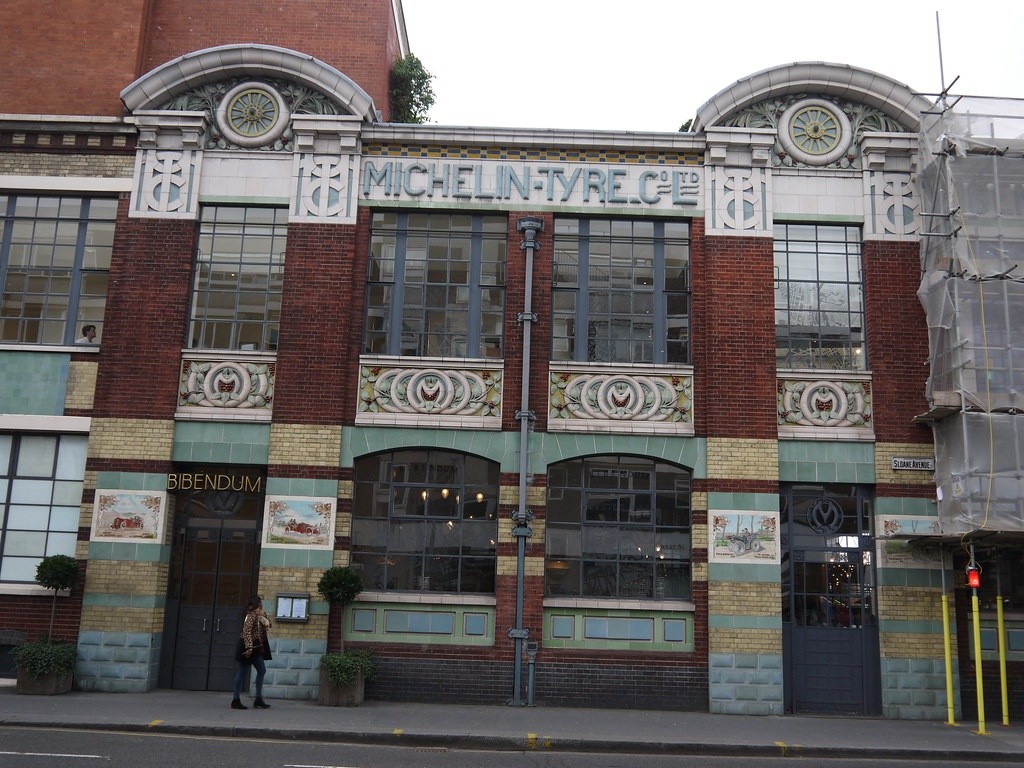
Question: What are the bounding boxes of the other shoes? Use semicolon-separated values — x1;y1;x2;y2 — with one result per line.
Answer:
231;698;247;709
254;697;270;708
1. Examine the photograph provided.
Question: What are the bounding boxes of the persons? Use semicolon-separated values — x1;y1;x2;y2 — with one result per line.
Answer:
231;595;272;709
